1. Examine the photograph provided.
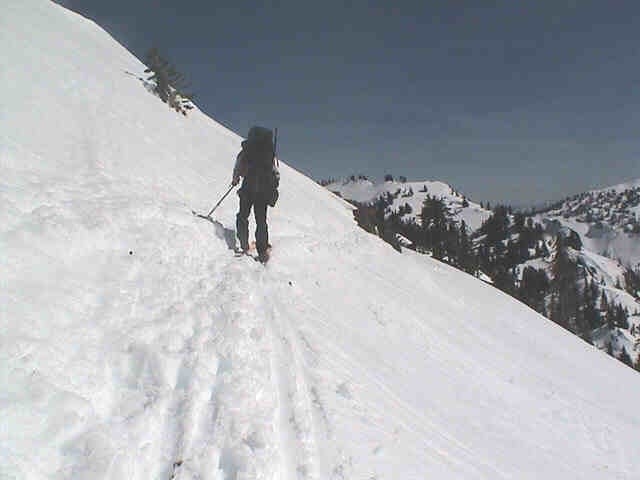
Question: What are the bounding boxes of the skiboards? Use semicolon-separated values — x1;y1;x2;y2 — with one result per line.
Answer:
208;184;272;256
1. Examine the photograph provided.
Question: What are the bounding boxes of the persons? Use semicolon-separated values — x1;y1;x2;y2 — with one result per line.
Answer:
231;127;279;262
